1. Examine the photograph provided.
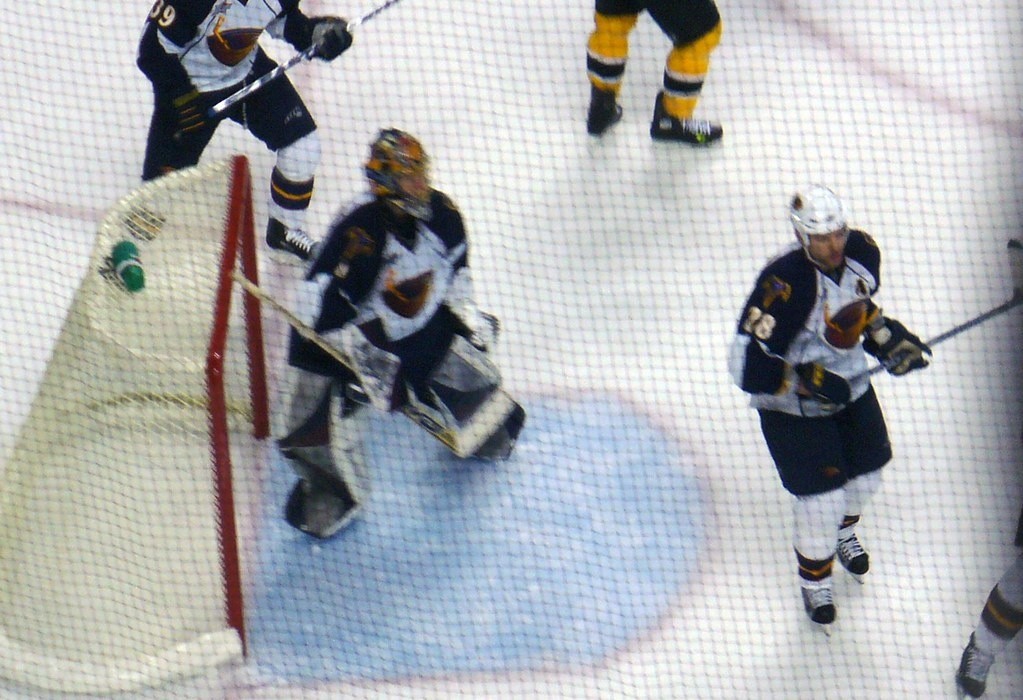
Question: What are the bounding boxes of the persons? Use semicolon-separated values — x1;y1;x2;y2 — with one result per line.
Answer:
587;0;724;143
126;0;353;261
276;126;525;539
728;188;932;623
955;510;1023;699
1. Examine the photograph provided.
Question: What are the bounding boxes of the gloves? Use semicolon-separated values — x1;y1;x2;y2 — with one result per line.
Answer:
794;362;851;411
861;317;933;375
163;84;213;145
285;8;352;61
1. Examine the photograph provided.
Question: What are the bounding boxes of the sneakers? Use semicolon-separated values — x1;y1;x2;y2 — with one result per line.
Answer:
264;217;322;267
650;91;723;144
955;631;995;700
794;545;836;637
587;83;622;134
835;516;869;584
98;234;143;297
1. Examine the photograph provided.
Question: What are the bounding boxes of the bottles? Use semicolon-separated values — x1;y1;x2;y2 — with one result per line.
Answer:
111;241;144;291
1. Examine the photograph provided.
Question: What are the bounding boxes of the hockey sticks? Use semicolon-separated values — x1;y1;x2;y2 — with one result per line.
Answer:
227;267;517;459
173;0;401;139
847;238;1023;382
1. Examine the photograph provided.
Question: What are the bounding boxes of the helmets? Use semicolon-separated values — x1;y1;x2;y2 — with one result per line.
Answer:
788;185;847;246
367;127;434;223
